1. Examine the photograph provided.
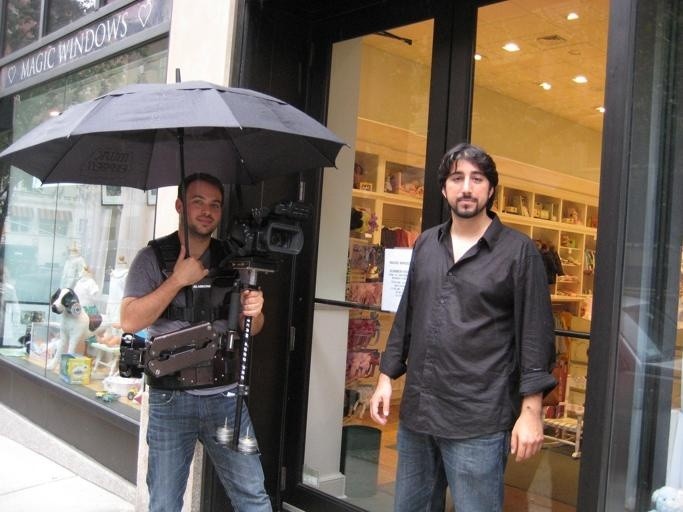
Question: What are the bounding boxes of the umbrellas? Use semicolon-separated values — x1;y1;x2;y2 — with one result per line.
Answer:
0;67;352;309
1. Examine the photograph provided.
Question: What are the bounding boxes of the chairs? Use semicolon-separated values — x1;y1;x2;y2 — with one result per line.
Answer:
539;374;587;458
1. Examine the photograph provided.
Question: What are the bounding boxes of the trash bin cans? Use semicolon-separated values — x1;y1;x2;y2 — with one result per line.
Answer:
341;425;382;498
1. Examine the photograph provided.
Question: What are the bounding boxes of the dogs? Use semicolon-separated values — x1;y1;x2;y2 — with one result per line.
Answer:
45;287;111;372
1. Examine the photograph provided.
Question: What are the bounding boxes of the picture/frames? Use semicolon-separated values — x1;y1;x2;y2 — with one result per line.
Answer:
0;301;52;348
101;184;124;206
147;187;157;206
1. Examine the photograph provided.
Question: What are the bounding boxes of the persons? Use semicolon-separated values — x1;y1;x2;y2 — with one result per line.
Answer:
120;173;271;511
370;144;558;512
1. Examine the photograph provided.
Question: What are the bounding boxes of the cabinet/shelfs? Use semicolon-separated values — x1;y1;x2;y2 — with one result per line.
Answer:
343;118;600;408
